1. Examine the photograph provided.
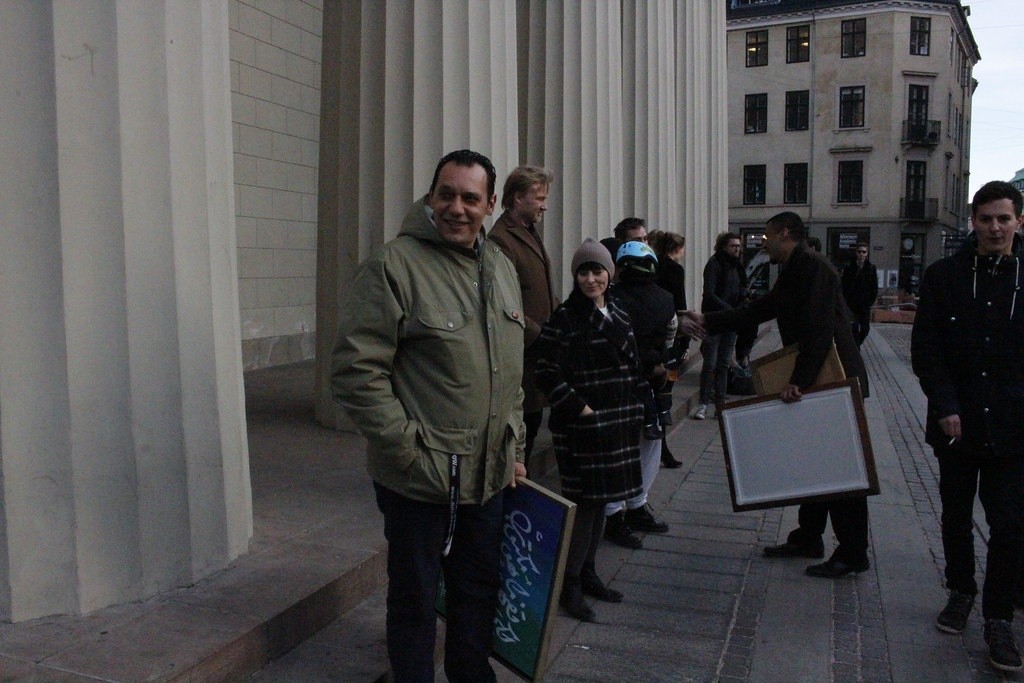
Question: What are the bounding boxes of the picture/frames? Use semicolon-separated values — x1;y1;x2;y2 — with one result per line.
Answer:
434;475;578;683
749;335;847;397
717;376;882;512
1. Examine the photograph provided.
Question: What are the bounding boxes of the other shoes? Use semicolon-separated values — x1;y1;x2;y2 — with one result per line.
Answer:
664;458;682;469
557;582;595;621
579;570;623;602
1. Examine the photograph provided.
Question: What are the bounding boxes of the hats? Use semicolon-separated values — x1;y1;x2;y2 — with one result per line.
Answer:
571;238;615;279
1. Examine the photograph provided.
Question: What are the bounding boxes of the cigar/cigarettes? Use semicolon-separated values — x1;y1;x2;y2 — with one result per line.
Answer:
949;436;956;445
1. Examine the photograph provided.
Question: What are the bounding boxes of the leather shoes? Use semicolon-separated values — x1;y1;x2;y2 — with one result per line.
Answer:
807;558;870;578
763;541;824;560
625;501;669;533
604;511;643;549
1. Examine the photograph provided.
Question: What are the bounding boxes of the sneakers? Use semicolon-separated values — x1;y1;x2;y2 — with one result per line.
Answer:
643;424;664;440
936;592;976;633
694;404;707;420
984;617;1023;671
658;412;673;426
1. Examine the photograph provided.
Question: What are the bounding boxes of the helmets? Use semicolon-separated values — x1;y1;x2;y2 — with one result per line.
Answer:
616;241;659;264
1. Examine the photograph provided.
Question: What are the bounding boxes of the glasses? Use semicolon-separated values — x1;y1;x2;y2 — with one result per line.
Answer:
857;250;867;254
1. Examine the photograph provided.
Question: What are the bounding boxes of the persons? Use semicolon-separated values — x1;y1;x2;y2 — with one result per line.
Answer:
328;148;526;683
910;179;1024;672
595;210;878;578
485;163;562;478
528;236;649;621
